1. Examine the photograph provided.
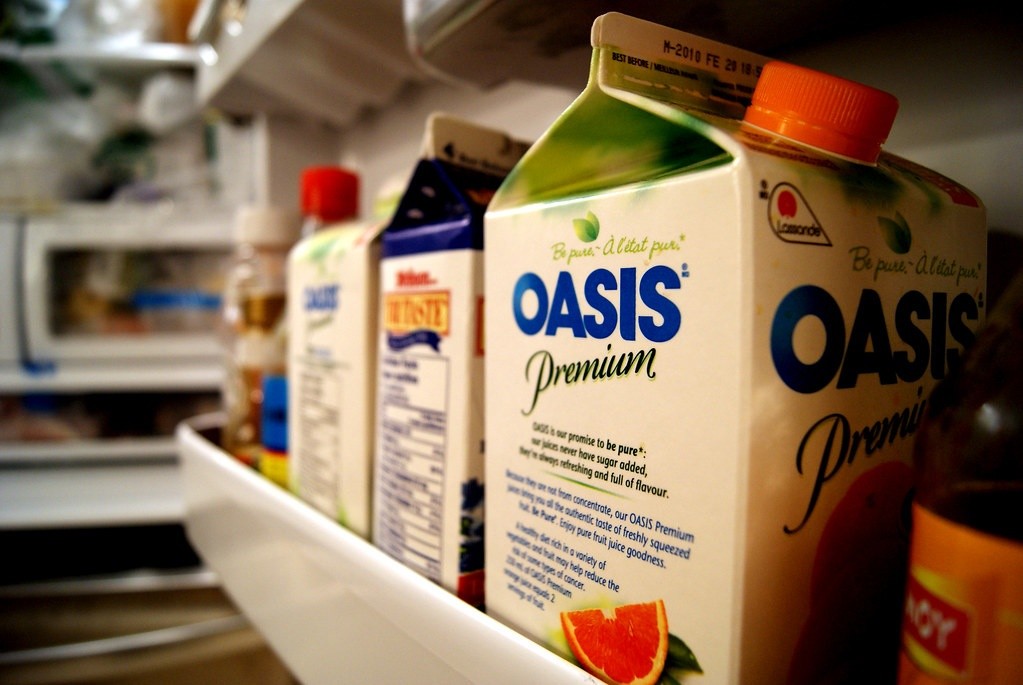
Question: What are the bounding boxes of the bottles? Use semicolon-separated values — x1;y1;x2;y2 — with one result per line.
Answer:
260;167;359;488
899;248;1023;685
220;209;299;469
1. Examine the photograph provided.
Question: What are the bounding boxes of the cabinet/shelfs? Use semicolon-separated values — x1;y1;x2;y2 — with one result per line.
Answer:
174;0;612;685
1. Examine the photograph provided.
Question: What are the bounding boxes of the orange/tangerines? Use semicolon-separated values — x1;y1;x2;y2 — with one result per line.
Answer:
560;598;669;685
